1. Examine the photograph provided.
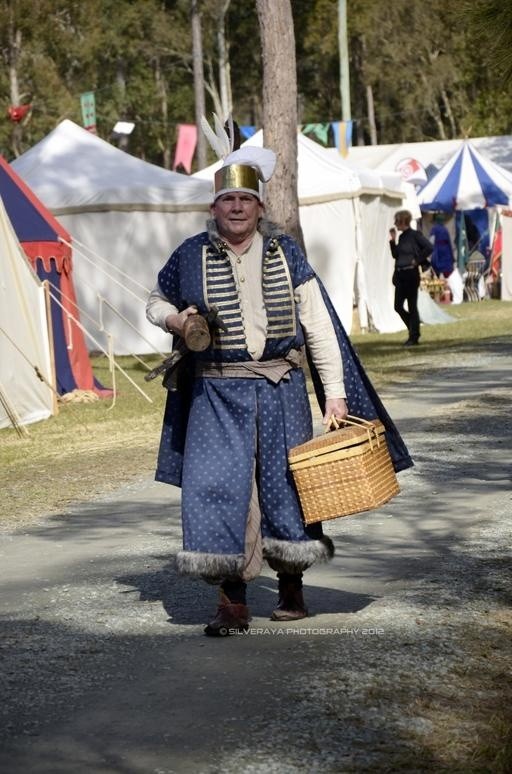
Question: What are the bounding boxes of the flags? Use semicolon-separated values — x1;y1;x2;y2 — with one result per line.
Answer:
78;90;98;134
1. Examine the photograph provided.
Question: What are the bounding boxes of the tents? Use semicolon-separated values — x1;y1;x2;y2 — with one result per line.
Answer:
0;119;511;432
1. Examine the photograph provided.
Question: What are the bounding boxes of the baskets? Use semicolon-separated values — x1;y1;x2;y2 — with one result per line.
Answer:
288;413;401;525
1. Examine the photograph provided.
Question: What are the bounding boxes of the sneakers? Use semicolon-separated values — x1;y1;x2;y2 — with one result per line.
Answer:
404;339;419;346
203;590;249;636
271;586;307;621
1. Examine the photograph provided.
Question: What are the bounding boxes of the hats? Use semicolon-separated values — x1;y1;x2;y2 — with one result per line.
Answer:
199;110;277;202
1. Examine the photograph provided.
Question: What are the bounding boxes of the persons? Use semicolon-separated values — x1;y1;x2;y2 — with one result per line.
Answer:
145;164;415;637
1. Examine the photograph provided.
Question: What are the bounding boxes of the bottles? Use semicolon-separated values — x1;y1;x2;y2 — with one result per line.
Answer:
183;304;211;352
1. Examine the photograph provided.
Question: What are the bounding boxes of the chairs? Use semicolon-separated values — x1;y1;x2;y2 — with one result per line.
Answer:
464;260;484;303
